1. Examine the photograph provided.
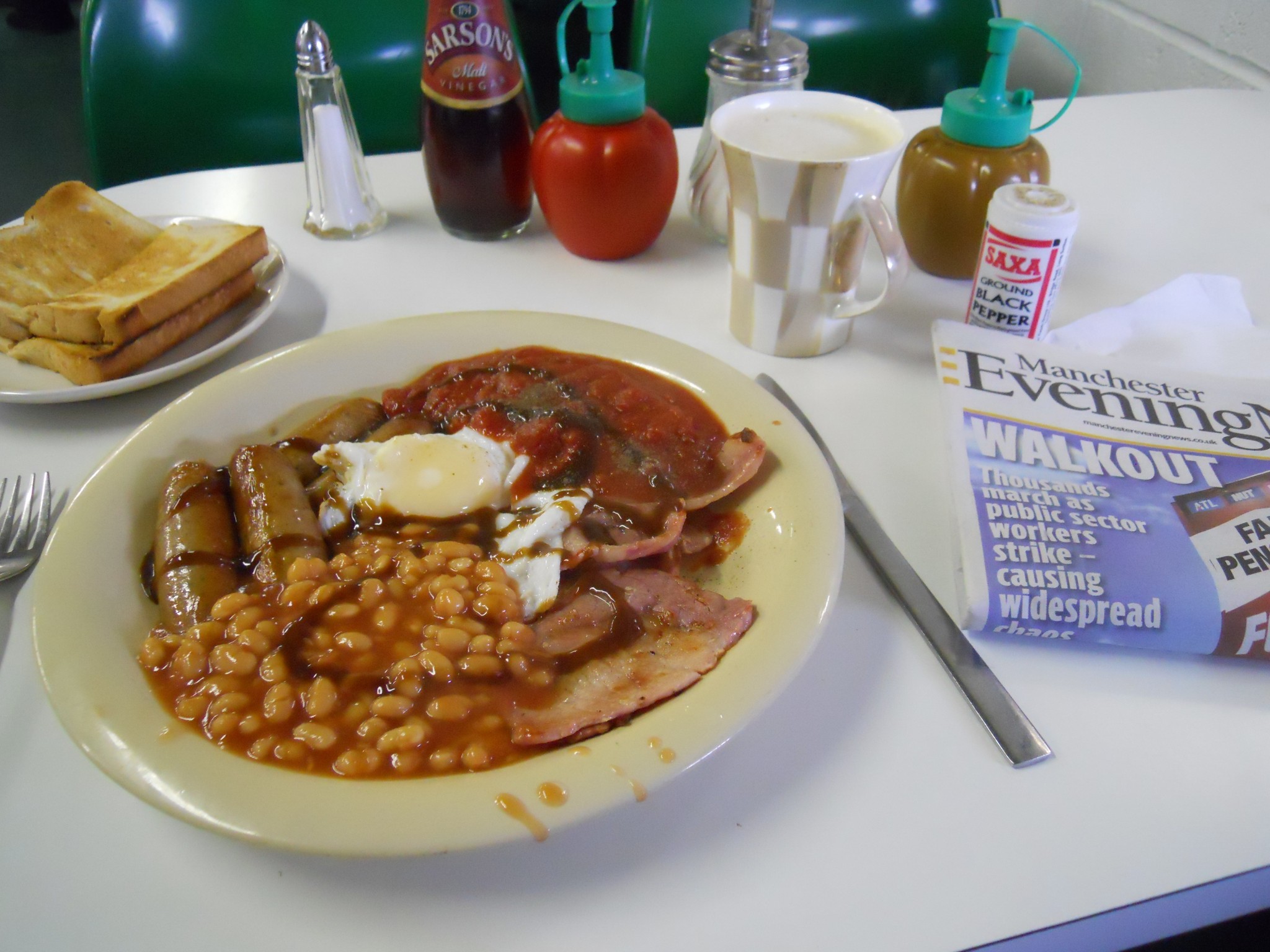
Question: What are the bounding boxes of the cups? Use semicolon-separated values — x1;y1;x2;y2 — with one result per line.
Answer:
708;89;907;358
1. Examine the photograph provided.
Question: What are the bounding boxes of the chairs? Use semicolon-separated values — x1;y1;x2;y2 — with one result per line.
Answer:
73;0;541;191
633;0;1004;128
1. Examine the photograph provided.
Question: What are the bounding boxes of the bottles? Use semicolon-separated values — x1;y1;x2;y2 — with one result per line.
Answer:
423;0;534;242
295;21;389;237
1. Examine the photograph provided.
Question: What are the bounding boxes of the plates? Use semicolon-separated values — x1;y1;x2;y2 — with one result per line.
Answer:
0;216;288;404
31;308;846;854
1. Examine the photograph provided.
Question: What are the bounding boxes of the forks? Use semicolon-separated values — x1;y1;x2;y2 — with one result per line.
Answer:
2;472;56;589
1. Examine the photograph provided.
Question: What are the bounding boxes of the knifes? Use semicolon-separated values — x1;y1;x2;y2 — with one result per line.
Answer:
756;368;1050;768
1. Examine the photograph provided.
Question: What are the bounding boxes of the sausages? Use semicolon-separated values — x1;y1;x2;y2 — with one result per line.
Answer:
153;393;431;642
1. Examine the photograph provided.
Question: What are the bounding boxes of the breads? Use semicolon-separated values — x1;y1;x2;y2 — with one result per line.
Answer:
1;180;275;384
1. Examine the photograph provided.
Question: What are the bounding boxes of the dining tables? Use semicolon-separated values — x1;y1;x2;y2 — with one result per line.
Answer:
1;90;1270;952
